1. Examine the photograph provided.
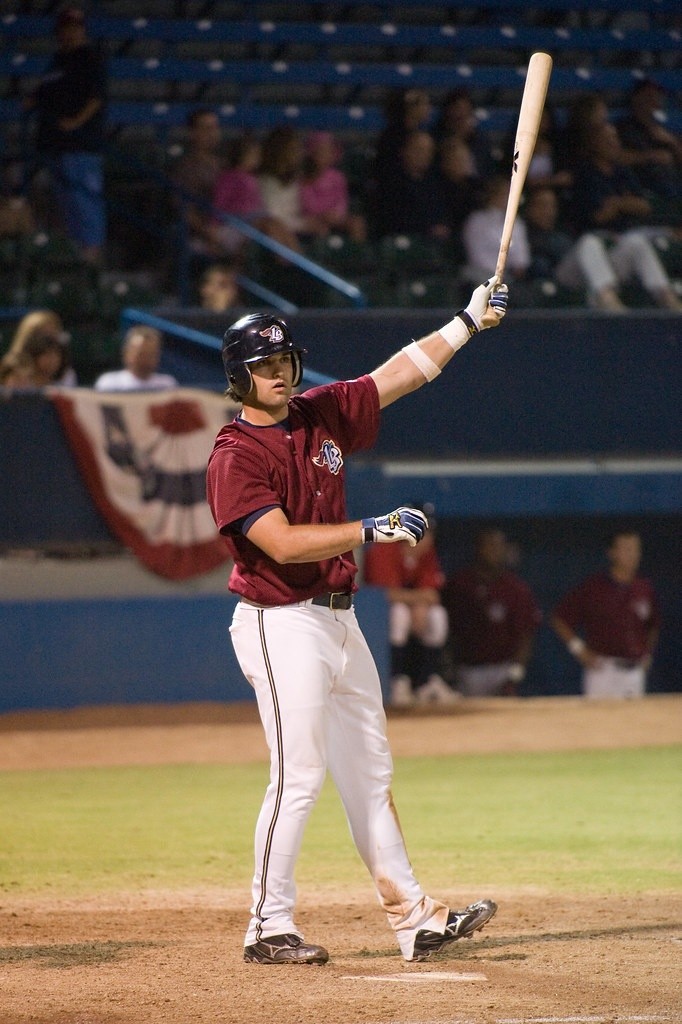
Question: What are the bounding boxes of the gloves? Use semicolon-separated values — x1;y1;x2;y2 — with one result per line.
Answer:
454;275;508;336
361;507;429;548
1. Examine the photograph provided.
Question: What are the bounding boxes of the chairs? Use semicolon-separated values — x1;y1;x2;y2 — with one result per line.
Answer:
0;0;682;379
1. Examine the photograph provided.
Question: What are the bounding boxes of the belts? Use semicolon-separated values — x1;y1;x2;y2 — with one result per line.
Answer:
311;591;353;612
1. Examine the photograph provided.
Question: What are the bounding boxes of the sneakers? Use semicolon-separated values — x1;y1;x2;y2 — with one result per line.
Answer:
244;934;328;967
413;898;499;962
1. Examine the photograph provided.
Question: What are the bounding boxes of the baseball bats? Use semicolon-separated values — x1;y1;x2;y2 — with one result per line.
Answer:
480;53;553;327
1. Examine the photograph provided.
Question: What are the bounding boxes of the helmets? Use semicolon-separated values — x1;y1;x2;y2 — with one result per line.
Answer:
221;314;307;398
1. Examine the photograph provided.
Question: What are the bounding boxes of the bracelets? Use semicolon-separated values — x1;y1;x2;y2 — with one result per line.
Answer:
566;637;583;654
438;319;469;353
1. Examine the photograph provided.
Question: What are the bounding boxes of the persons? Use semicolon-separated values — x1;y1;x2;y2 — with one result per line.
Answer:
93;325;177;394
21;5;109;267
0;312;63;387
442;523;539;698
206;275;508;965
176;107;366;290
551;531;658;699
366;80;682;314
367;497;465;705
